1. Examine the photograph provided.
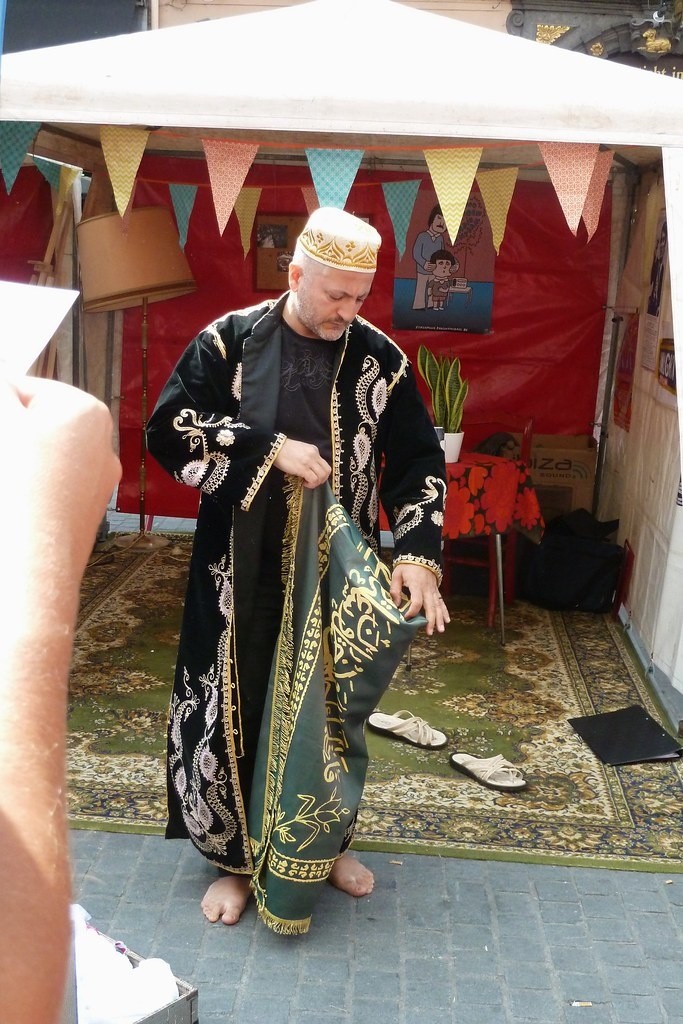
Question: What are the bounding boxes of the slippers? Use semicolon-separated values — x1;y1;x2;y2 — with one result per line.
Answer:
366;710;448;749
450;752;527;792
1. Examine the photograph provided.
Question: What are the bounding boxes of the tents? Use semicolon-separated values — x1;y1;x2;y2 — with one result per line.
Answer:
0;0;683;737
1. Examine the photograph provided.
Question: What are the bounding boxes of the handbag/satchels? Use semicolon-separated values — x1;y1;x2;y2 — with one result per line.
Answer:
523;529;627;614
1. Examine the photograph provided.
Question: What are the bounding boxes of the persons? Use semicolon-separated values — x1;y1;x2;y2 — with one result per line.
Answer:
0;367;123;1024
140;203;454;928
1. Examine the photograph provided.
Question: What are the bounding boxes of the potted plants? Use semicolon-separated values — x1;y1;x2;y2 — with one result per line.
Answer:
417;344;469;463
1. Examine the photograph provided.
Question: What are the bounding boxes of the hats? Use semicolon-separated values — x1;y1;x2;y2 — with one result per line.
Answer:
297;207;382;273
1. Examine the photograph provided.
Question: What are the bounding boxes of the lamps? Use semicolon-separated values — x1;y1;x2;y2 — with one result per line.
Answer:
76;206;198;549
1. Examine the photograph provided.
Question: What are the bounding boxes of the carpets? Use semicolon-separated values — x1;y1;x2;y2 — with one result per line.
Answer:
62;530;683;874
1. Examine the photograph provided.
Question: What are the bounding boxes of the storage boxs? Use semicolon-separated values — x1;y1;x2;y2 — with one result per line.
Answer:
501;433;598;525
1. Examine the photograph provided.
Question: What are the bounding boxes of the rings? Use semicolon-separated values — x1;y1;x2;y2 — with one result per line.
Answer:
438;596;443;599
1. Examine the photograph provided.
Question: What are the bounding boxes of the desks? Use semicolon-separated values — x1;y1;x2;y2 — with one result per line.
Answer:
377;450;547;630
